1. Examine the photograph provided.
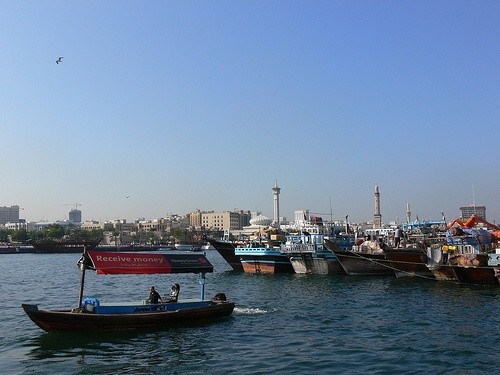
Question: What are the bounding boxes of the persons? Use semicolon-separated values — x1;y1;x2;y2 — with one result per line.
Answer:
147;286;164;311
442;242;449;264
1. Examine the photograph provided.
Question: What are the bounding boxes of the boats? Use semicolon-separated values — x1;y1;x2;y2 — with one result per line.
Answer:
205;208;500;288
21;244;235;335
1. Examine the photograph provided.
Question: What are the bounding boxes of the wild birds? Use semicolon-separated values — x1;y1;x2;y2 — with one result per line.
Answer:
55;56;64;64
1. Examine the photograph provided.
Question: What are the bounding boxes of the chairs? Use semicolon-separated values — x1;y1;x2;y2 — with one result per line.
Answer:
163;283;180;303
